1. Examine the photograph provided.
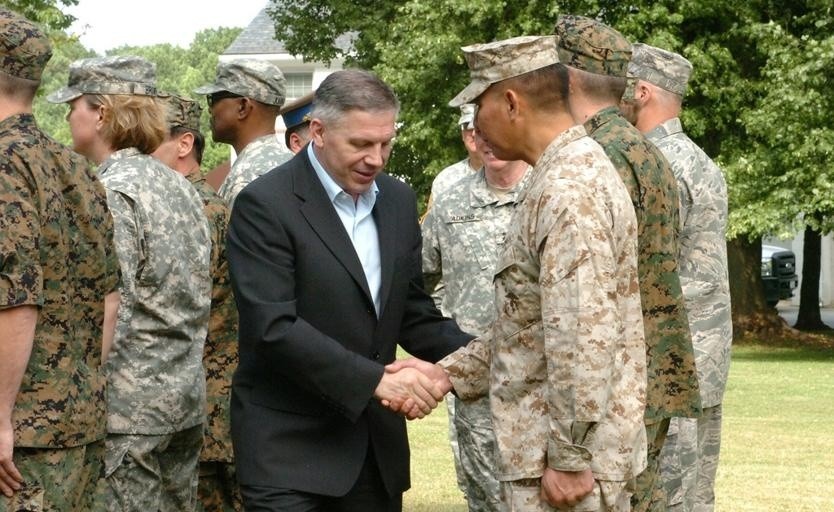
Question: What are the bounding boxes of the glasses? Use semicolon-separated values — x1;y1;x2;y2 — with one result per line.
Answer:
207;94;243;106
461;123;473;130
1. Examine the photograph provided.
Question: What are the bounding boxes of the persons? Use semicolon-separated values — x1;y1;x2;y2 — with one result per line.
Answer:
42;51;212;511
424;104;481;499
148;88;243;511
0;6;126;511
278;91;316;155
626;39;735;511
191;56;295;211
618;62;640;126
417;104;534;512
381;29;648;512
551;14;703;512
222;68;476;512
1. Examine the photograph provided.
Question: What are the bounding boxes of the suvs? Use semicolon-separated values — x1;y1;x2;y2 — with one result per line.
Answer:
758;245;798;313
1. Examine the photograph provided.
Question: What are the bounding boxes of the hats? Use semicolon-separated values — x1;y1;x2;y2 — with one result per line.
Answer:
158;90;203;131
456;103;476;125
280;91;317;130
447;35;560;108
628;43;692;96
0;7;53;81
550;14;633;77
45;56;157;103
193;58;286;106
